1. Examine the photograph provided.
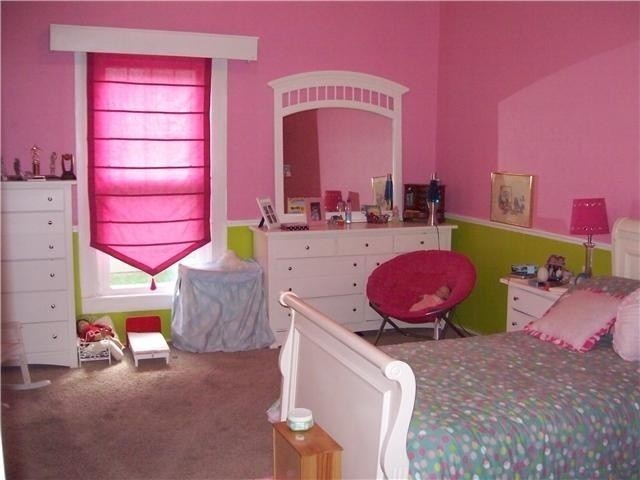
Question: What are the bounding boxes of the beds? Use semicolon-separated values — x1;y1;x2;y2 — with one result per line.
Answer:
265;216;640;480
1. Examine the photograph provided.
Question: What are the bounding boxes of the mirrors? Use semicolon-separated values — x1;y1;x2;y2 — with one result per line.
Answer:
267;69;411;225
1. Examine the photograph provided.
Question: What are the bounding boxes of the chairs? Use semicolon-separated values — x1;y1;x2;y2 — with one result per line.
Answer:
365;248;473;344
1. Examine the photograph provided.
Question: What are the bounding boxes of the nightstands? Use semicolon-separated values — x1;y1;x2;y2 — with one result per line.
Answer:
499;276;568;331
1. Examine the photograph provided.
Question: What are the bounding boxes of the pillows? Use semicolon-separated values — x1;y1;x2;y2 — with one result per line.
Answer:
612;289;640;363
522;285;628;353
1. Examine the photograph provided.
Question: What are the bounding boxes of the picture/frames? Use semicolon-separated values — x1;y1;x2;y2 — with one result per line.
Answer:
304;196;326;224
489;170;533;229
256;196;279;229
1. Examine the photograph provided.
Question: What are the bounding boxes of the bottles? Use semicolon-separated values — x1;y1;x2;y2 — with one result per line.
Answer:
382;172;393;212
406;188;414;208
344;198;352;224
425;170;440;227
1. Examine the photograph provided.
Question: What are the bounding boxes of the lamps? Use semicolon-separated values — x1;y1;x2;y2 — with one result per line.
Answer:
570;198;609;276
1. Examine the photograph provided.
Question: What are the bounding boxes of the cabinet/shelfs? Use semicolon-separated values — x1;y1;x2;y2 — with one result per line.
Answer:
272;423;342;480
0;180;79;370
247;225;459;348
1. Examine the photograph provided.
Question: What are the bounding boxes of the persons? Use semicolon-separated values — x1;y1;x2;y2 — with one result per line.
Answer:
410;285;450;310
76;318;126;361
497;193;526;217
